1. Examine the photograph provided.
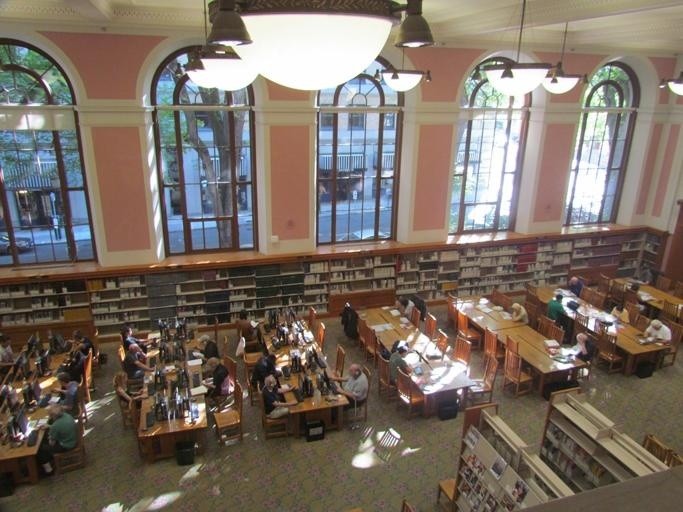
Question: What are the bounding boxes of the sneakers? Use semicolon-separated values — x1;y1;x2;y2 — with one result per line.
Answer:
41;470;55;476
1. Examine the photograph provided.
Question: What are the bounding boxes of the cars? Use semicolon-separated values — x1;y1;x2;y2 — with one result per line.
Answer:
1;234;32;255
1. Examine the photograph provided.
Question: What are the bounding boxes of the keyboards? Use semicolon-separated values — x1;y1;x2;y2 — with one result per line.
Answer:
40;394;51;408
282;366;289;377
293;388;305;402
150;356;156;368
188;330;195;339
148;383;155;395
27;428;38;446
192;373;200;387
192;402;199;418
188;350;195;360
264;323;270;333
147;412;154;427
272;337;281;349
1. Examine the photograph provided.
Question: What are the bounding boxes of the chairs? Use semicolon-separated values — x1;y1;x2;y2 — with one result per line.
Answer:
437;478;457;506
113;303;373;469
401;498;413;512
353;276;680;416
1;329;101;404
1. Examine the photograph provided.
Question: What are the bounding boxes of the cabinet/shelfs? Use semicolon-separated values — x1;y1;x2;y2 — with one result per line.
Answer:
0;223;669;352
451;386;671;511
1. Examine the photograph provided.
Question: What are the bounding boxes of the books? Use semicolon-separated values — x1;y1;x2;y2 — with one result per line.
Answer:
455;422;618;512
0;233;662;328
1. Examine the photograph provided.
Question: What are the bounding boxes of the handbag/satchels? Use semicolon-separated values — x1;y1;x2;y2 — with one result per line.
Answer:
391;340;408;355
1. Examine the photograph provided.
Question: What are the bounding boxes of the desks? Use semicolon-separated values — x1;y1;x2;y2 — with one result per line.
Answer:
0;376;62;485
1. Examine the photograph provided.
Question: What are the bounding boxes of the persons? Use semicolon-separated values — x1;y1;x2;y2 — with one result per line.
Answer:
0;325;231;479
262;374;299;436
575;333;595;362
387;345;411;387
644;318;671;344
250;355;283;390
327;363;368;423
234;309;264;343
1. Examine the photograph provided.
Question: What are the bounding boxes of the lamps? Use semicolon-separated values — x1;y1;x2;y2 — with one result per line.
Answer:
183;1;436;93
470;0;683;98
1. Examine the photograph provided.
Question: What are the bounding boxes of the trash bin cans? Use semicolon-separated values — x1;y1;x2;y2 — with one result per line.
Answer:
636;360;652;378
175;441;194;466
438;401;458;420
305;420;324;443
0;471;15;497
543;380;579;401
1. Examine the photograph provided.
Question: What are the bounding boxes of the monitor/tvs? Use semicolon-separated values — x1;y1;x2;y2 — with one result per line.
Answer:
265;307;332;397
0;306;68;435
154;316;190;421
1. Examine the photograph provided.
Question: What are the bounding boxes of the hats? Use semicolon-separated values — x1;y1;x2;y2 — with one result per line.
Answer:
347;364;360;372
197;335;209;342
